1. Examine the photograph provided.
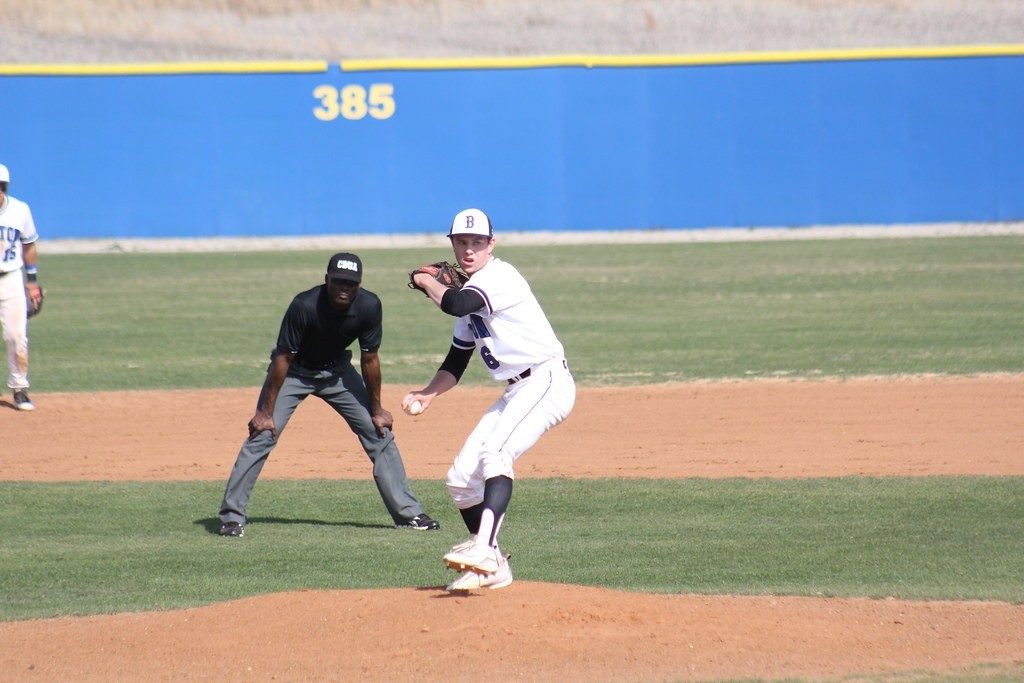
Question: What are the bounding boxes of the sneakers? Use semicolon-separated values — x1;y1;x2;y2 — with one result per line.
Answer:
443;534;498;576
394;512;440;531
446;553;513;590
13;391;34;410
219;521;244;537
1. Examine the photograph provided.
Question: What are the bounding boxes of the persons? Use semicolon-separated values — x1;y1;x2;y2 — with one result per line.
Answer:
401;208;576;593
218;252;440;537
0;163;43;411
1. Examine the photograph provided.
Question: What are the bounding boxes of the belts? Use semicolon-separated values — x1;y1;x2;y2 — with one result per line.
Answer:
508;359;565;385
0;272;8;277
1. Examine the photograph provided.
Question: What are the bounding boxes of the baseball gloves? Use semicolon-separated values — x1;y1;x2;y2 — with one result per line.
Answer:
408;261;469;297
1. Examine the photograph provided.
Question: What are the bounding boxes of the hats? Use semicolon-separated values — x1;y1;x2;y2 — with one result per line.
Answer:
0;164;10;183
446;208;493;238
327;253;362;283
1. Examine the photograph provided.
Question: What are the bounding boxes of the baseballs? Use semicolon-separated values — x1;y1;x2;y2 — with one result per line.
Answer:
403;400;422;416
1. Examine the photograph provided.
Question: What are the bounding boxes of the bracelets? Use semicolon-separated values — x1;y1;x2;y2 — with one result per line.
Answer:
25;264;37;275
27;273;36;282
